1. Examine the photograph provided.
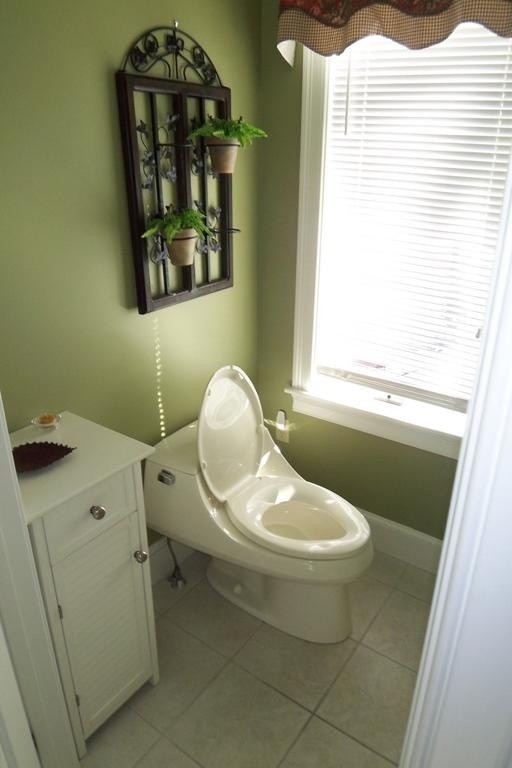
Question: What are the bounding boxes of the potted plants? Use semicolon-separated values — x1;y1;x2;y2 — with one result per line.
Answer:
185;112;268;176
144;208;210;268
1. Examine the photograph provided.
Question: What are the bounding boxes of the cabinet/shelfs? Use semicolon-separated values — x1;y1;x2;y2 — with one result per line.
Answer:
9;409;160;761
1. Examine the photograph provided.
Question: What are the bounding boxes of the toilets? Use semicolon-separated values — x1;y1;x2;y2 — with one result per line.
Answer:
142;365;374;645
